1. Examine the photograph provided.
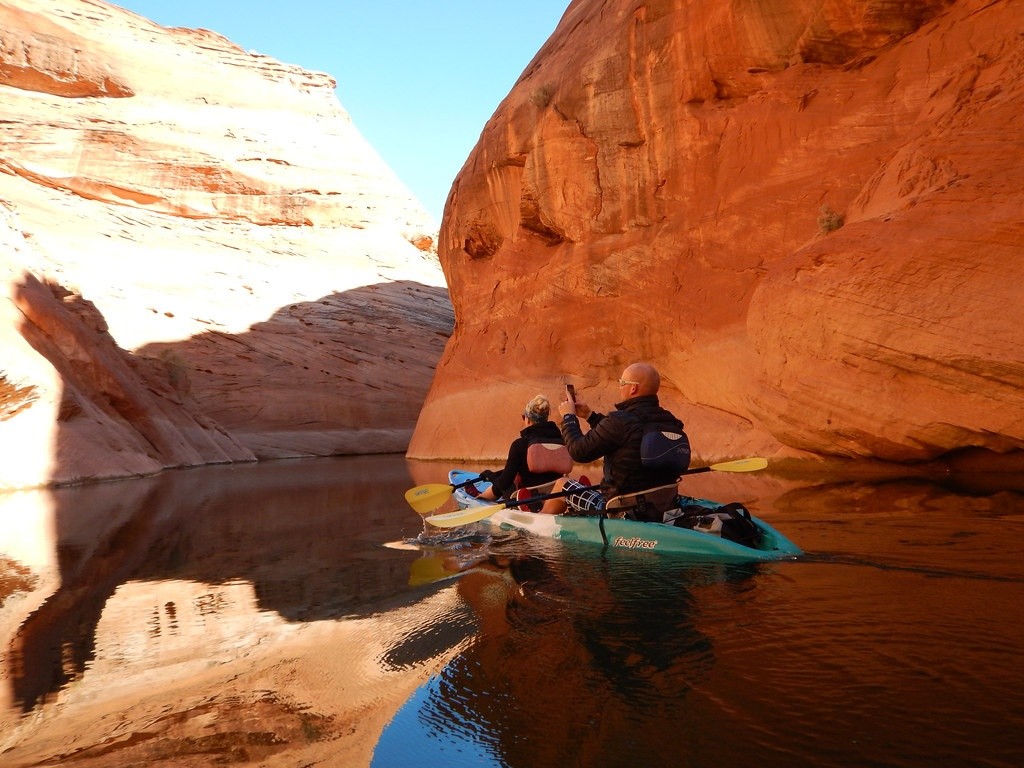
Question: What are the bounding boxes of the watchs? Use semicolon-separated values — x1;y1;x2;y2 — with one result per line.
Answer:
563;413;576;420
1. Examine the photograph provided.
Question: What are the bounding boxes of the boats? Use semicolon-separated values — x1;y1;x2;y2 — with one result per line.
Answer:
445;466;805;564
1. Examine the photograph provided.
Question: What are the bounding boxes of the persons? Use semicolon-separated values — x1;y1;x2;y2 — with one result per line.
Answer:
463;395;565;514
518;362;691;518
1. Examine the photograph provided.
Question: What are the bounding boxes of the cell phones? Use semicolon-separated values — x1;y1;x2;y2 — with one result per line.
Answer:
566;384;577;403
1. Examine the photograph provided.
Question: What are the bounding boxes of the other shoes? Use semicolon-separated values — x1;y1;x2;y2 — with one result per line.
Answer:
578;475;592;489
516;488;542;513
464;479;481;498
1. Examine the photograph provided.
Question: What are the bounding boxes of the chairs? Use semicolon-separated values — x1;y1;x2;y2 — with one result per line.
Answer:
510;480;556;513
605;476;682;520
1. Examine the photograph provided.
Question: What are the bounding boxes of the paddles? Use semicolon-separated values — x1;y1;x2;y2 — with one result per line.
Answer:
379;533;499;588
425;457;768;529
403;468;506;514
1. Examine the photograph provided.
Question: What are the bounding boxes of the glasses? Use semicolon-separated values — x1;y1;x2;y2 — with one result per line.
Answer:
522;414;529;421
619;379;638;386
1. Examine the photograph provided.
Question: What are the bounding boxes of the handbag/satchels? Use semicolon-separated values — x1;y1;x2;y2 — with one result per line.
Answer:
662;502;765;549
